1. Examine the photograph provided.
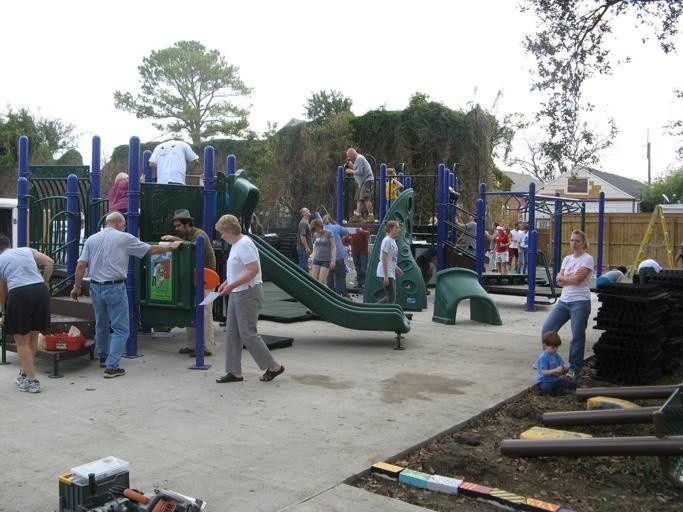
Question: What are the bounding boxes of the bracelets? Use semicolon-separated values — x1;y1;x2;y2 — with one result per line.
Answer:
73;284;81;289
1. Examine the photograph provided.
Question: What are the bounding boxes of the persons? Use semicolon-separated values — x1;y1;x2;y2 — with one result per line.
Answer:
298;208;369;298
0;234;54;393
376;221;403;304
385;168;403;210
148;140;200;185
109;172;146;212
596;266;626;285
417;248;437;295
533;229;594;378
454;215;477;255
485;213;529;275
537;332;577;395
638;259;662;284
215;213;284;383
70;211;184;378
161;209;216;357
345;150;375;222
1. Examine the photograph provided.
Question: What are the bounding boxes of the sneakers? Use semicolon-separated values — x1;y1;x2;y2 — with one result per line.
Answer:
15;368;28;386
104;368;128;378
260;364;284;382
179;346;197;354
18;379;41;393
566;369;580;379
216;371;244;383
100;355;107;368
364;215;375;223
349;214;363;223
191;349;212;357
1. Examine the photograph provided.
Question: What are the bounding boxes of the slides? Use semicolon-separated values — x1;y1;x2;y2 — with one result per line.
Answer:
244;229;410;335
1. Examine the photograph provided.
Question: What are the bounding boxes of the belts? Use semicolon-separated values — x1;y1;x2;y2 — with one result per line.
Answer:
90;279;126;285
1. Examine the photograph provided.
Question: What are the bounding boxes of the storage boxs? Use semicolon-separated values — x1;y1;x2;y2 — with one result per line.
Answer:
46;333;85;351
59;467;129;512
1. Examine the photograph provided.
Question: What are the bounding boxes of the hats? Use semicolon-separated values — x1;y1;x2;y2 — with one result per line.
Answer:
170;209;195;222
